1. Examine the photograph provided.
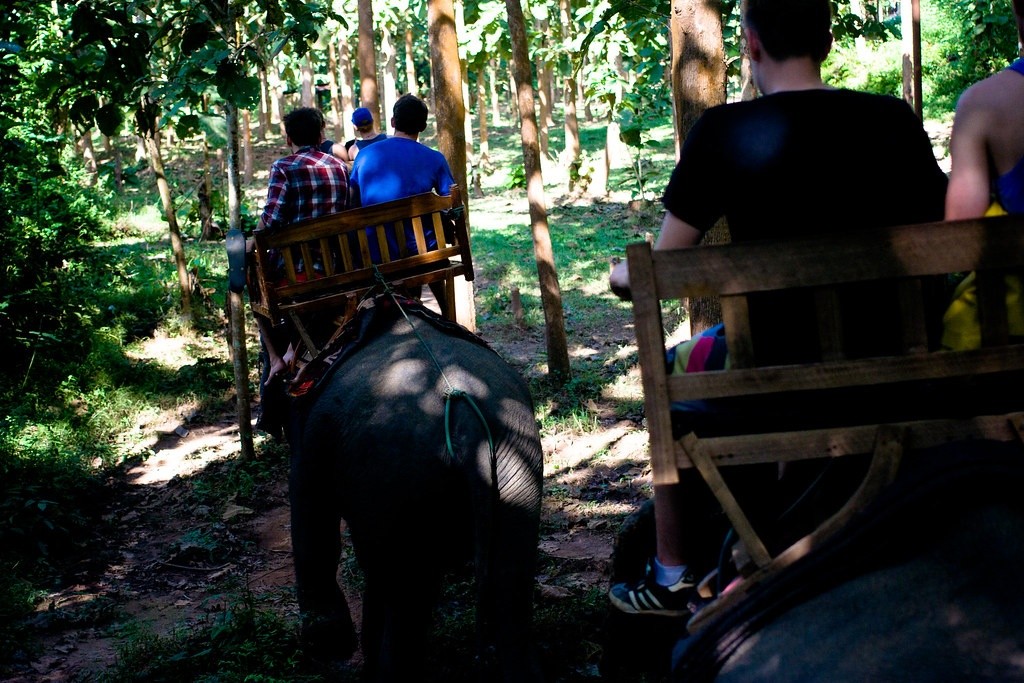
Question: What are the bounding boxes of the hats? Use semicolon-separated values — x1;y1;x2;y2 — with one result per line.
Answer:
352;107;372;127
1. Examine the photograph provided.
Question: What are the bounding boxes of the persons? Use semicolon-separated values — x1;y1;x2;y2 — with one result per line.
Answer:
244;107;349;383
946;1;1024;351
610;1;951;616
318;112;349;161
346;95;457;319
345;107;388;162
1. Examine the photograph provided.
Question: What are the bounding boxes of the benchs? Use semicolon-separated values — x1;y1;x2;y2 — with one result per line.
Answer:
249;184;474;360
627;212;1023;635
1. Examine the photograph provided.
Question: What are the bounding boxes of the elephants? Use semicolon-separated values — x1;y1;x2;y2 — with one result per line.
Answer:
681;555;1024;683
257;264;545;683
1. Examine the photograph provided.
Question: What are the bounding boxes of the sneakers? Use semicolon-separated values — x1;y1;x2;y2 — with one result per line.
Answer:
607;558;698;615
